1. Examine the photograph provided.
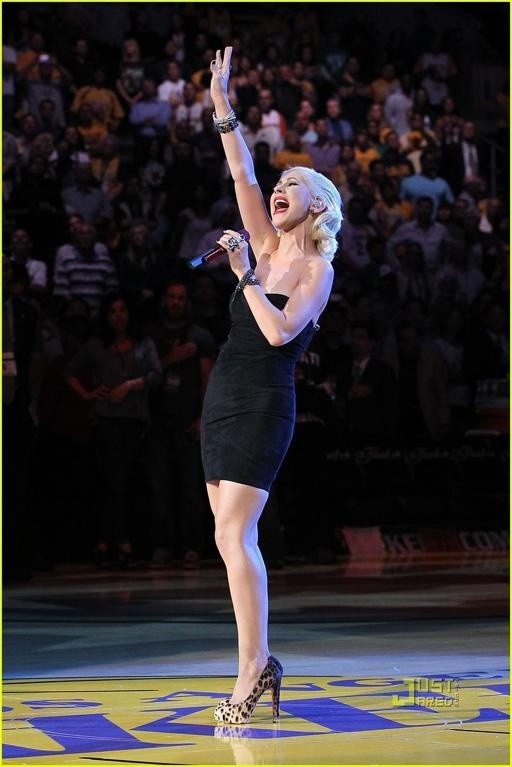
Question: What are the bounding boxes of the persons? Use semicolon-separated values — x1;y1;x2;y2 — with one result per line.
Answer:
189;46;345;727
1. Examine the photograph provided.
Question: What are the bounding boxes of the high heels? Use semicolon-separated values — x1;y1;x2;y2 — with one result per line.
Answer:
214;655;283;724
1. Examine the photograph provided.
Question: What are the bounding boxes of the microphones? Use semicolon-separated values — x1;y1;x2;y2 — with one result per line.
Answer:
188;229;251;269
